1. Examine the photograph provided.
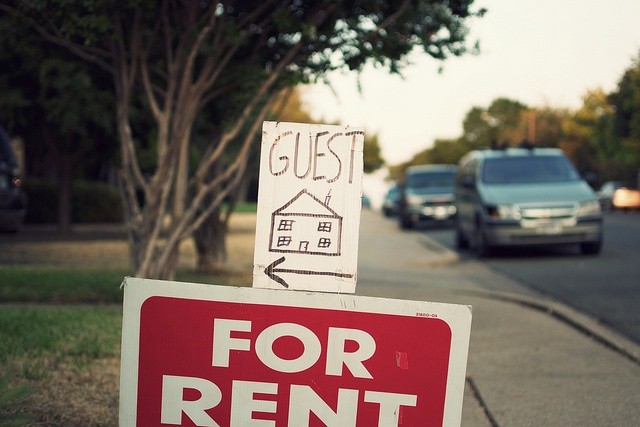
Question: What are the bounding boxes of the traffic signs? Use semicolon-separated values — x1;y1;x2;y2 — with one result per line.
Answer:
114;277;475;427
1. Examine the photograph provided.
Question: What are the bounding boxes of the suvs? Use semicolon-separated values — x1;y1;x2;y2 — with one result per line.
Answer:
454;141;602;256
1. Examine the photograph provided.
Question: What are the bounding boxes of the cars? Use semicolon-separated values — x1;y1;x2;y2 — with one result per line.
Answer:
382;186;401;219
400;163;460;232
